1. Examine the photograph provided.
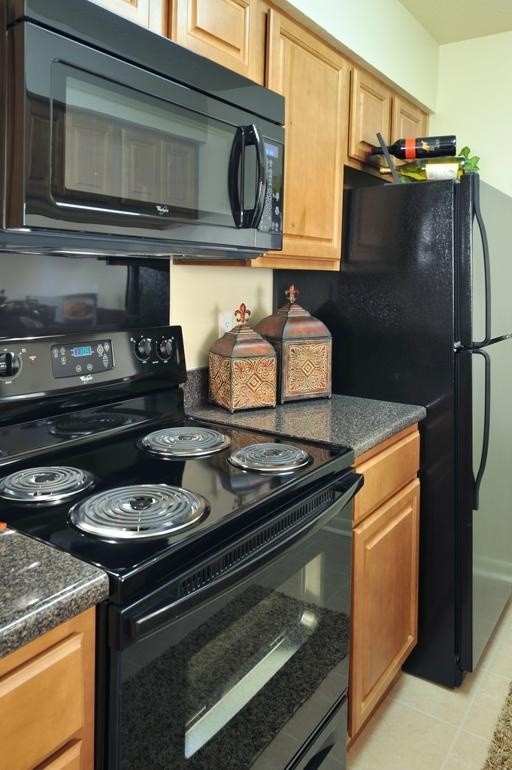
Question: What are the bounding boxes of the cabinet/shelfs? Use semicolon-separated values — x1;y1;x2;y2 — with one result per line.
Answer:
347;423;421;753
345;55;435;189
1;605;96;770
173;2;354;273
93;0;266;89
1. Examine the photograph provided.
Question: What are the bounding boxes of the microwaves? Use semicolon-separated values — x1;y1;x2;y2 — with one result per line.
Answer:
2;0;292;265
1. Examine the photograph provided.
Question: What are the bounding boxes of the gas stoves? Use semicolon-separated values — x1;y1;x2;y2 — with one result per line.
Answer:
0;424;353;606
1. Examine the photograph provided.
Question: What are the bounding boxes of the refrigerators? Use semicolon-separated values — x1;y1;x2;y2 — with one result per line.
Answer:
271;173;510;691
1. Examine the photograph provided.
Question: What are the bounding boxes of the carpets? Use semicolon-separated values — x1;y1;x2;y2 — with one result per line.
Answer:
481;681;512;770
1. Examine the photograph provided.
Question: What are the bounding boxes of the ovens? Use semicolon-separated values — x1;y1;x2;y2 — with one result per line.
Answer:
98;461;364;770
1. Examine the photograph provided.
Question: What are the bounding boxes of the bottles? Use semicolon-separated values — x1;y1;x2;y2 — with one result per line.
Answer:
378;157;464;182
367;135;456;160
